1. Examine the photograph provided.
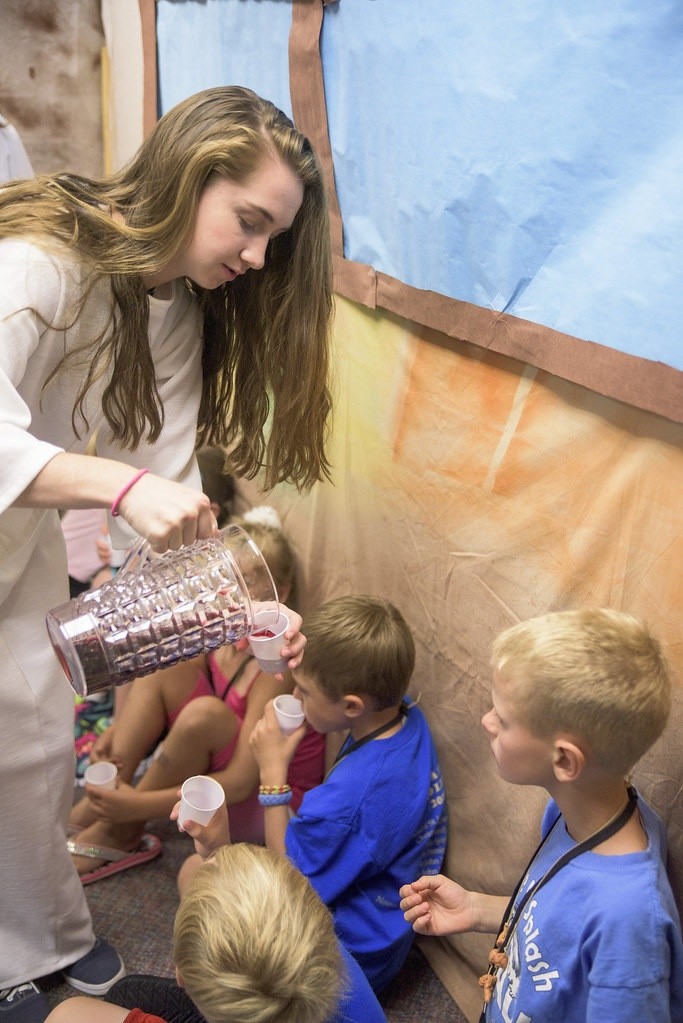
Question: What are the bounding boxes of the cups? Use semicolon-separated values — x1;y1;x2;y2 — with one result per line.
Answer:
246;610;290;674
273;694;306;736
84;760;118;790
177;775;226;831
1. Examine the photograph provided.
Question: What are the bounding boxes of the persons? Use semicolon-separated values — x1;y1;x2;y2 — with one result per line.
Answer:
0;86;333;1023
399;610;683;1023
41;471;346;1023
249;594;448;992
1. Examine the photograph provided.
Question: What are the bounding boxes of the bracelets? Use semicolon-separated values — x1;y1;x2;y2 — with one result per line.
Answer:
258;792;293;807
112;468;149;517
258;784;293;795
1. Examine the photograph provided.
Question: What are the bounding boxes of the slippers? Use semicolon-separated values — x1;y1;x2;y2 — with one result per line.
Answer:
68;825;161;886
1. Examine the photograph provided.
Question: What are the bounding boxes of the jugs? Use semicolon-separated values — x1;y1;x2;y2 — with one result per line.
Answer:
44;509;281;699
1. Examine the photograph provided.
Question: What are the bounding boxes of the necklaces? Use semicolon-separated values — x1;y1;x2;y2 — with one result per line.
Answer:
479;791;629;1006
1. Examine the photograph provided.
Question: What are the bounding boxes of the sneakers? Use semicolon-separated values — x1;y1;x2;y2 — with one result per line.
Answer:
60;936;126;996
0;982;52;1023
